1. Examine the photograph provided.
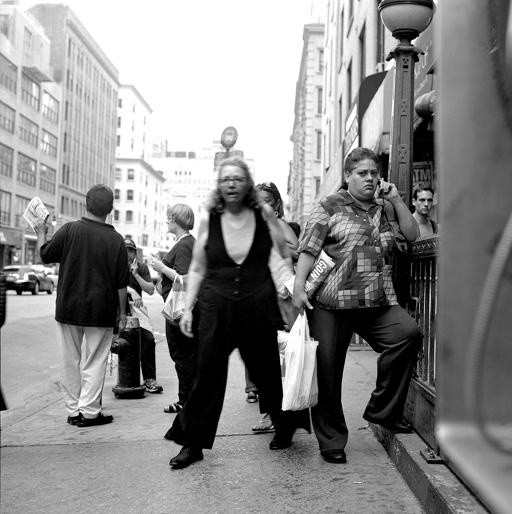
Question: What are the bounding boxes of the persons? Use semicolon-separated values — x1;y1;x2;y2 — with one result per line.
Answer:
111;293;164;394
292;147;422;466
287;222;301;240
124;239;156;310
246;364;260;405
169;157;292;472
412;185;439;238
151;204;198;414
35;183;131;428
252;182;300;432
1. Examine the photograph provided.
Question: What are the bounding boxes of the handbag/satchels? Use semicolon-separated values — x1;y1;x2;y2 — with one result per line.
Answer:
161;273;198;322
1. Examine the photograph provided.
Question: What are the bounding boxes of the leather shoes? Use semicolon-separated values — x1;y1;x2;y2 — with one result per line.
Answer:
67;413;81;426
247;391;257;403
270;428;296;450
77;412;113;427
169;448;203;468
363;410;414;434
323;448;347;464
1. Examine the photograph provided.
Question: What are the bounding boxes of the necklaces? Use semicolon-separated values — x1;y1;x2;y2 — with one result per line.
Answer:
220;209;252;231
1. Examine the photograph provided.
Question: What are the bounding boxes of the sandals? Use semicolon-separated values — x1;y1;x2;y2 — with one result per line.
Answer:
164;402;183;413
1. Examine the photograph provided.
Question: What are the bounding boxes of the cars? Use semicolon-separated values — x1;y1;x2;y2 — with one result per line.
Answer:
0;263;54;295
44;266;58;287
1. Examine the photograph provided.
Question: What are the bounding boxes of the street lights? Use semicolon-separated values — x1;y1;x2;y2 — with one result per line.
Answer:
378;0;440;308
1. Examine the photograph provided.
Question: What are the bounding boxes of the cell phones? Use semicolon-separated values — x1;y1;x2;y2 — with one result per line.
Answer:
133;257;137;264
377;177;383;197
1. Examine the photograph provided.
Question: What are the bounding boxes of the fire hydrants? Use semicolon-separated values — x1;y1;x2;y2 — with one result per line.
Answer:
107;315;146;400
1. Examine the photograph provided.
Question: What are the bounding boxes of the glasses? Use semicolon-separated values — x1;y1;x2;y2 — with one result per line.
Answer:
217;177;247;187
255;181;273;188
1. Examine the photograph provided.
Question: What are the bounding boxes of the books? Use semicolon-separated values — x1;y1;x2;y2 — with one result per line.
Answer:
23;196;52;237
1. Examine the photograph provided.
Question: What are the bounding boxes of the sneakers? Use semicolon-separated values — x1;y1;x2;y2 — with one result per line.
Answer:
142;378;163;393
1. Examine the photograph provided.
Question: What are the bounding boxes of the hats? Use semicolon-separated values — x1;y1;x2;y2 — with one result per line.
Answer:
124;239;137;250
